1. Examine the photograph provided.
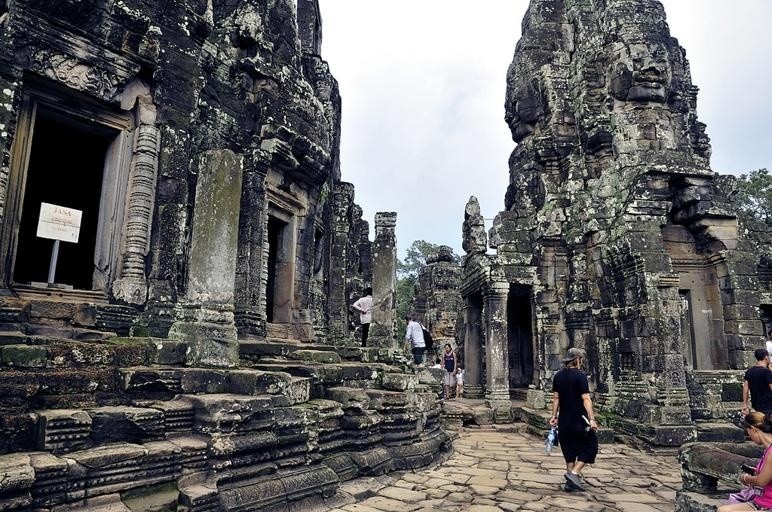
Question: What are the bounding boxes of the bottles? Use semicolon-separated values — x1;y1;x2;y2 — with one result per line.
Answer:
545;426;559;454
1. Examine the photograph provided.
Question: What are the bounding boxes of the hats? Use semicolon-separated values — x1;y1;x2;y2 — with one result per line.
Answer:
561;348;582;362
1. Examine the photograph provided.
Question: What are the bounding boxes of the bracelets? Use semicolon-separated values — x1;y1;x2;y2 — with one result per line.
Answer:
551;415;558;419
743;472;749;484
589;418;598;424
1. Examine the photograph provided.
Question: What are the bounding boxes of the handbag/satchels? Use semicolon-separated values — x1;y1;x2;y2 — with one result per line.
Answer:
728;488;756;505
417;321;433;349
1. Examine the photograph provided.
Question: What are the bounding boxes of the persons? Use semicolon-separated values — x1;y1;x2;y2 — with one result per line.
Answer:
432;358;444;370
764;329;772;372
401;313;430;365
439;343;457;399
454;367;466;398
740;347;772;417
350;286;373;348
710;411;772;512
429;360;435;369
437;306;460;337
547;347;600;495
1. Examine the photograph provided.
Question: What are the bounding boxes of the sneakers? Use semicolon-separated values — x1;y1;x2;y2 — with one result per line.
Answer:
564;469;586;492
